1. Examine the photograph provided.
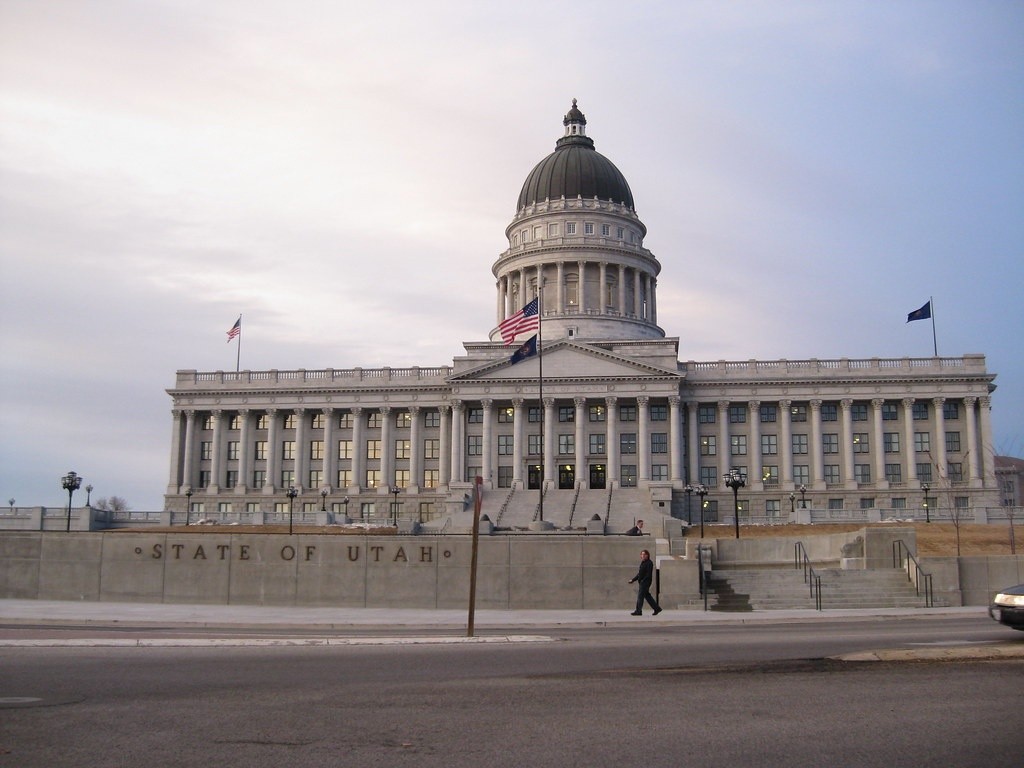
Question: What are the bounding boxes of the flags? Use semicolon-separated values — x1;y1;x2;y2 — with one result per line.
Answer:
908;301;932;322
499;297;540;345
227;318;241;343
510;334;537;365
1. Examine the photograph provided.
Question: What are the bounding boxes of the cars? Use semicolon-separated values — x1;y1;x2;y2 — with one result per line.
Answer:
988;580;1024;636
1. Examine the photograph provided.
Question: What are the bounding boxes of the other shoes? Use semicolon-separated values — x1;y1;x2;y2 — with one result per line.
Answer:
631;611;642;615
652;607;662;615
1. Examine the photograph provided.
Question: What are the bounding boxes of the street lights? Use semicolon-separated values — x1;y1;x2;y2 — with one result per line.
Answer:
392;485;402;525
61;471;83;532
186;488;193;526
695;484;710;539
921;484;932;523
684;484;695;525
286;486;298;535
722;467;748;538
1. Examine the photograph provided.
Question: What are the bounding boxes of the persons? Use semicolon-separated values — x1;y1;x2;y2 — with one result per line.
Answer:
628;550;663;616
625;520;644;537
